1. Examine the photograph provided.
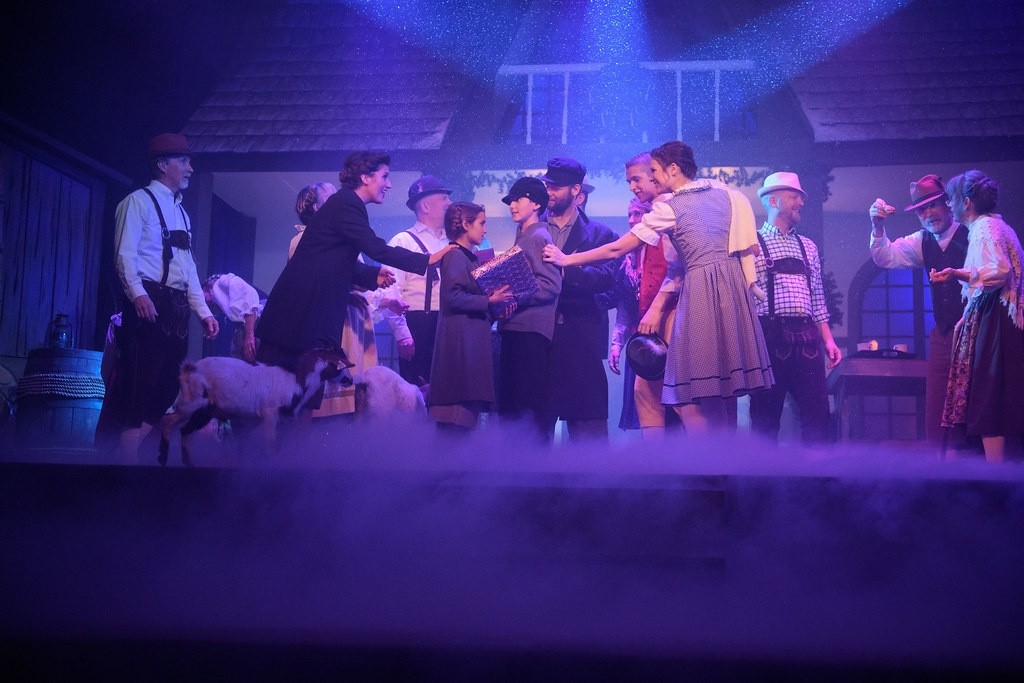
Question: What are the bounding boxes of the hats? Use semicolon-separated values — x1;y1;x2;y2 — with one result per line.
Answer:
502;177;549;214
406;174;452;210
148;132;199;160
904;174;947;212
626;331;671;381
541;157;586;184
581;174;595;194
757;171;808;199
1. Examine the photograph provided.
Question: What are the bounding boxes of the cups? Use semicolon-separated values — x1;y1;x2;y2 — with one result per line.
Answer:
857;343;872;352
893;344;909;352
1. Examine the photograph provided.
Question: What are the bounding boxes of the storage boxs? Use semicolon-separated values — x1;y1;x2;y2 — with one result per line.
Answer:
471;245;537;316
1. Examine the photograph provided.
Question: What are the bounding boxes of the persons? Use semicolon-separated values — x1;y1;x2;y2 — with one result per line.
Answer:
929;170;1024;465
253;150;459;440
380;175;452;387
750;171;842;452
870;174;969;462
202;272;269;366
540;140;776;450
498;176;562;456
426;202;517;449
93;134;220;466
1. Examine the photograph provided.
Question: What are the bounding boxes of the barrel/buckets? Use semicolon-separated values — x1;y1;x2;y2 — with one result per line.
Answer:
17;347;106;463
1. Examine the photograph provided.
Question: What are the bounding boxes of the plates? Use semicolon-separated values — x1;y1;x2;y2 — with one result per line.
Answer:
626;332;670;379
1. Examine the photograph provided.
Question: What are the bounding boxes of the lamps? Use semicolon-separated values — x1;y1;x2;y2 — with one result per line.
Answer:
44;312;75;351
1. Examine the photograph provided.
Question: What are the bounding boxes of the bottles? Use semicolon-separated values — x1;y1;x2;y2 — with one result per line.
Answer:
869;340;878;351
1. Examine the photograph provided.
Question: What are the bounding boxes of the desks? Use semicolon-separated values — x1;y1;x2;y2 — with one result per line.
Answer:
825;358;928;443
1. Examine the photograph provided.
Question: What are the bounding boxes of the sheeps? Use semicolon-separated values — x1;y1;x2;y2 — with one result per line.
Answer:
161;338;356;465
354;366;432;454
